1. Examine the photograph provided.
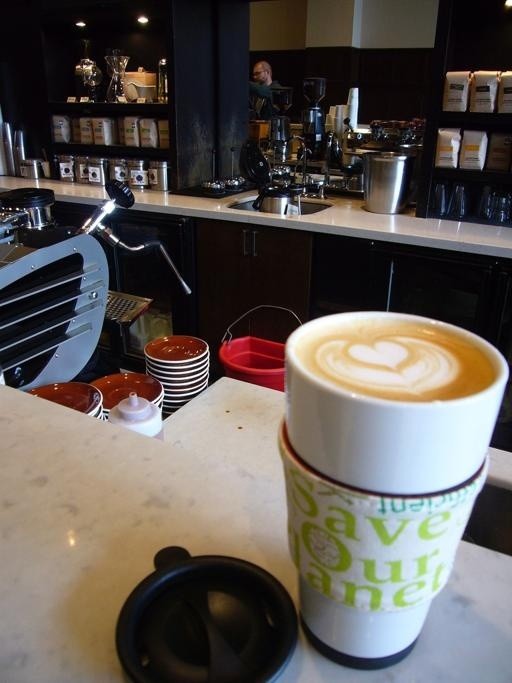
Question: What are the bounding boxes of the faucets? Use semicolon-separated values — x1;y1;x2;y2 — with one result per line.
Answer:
290;134;311;188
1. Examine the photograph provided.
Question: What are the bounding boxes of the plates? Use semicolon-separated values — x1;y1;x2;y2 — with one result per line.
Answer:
28;380;107;423
144;333;212;418
91;369;164;419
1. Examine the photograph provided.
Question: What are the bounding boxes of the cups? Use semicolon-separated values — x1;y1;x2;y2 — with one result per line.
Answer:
325;87;359;130
276;308;511;673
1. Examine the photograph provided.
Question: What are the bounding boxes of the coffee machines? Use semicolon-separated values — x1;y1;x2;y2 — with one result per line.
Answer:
268;84;295;159
302;76;326;160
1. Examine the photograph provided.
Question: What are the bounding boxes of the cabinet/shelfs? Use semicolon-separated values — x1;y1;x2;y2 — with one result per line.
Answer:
45;99;172;161
430;110;512;186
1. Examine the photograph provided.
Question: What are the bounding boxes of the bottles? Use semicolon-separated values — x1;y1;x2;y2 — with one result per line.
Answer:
105;390;163;440
434;182;510;226
51;152;165;191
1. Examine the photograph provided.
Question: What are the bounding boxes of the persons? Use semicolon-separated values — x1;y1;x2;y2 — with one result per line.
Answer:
252;60;286;119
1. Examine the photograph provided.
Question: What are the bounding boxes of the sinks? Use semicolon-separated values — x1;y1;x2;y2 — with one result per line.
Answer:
227;198;334;216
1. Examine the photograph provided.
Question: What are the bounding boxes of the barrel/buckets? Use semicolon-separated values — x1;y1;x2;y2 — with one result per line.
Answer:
217;304;303;395
